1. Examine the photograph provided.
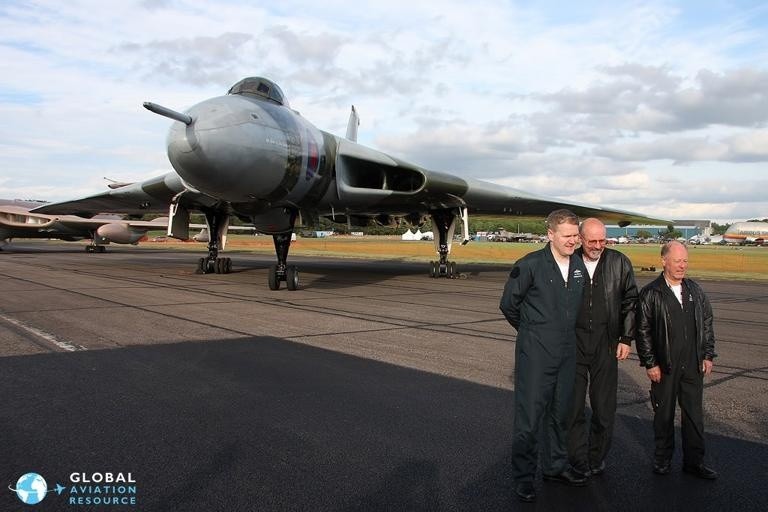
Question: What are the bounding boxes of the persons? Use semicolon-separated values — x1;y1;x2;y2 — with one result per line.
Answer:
498;208;590;504
565;217;640;479
635;241;719;482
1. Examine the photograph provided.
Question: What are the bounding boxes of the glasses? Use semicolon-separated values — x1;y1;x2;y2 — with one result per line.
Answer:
588;239;607;246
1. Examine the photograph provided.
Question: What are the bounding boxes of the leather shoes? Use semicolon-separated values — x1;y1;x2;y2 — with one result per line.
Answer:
514;481;536;502
682;462;721;479
653;459;673;474
543;459;607;486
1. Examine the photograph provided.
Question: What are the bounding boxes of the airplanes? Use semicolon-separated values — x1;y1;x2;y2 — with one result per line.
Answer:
0;198;257;253
27;76;676;290
722;222;767;247
